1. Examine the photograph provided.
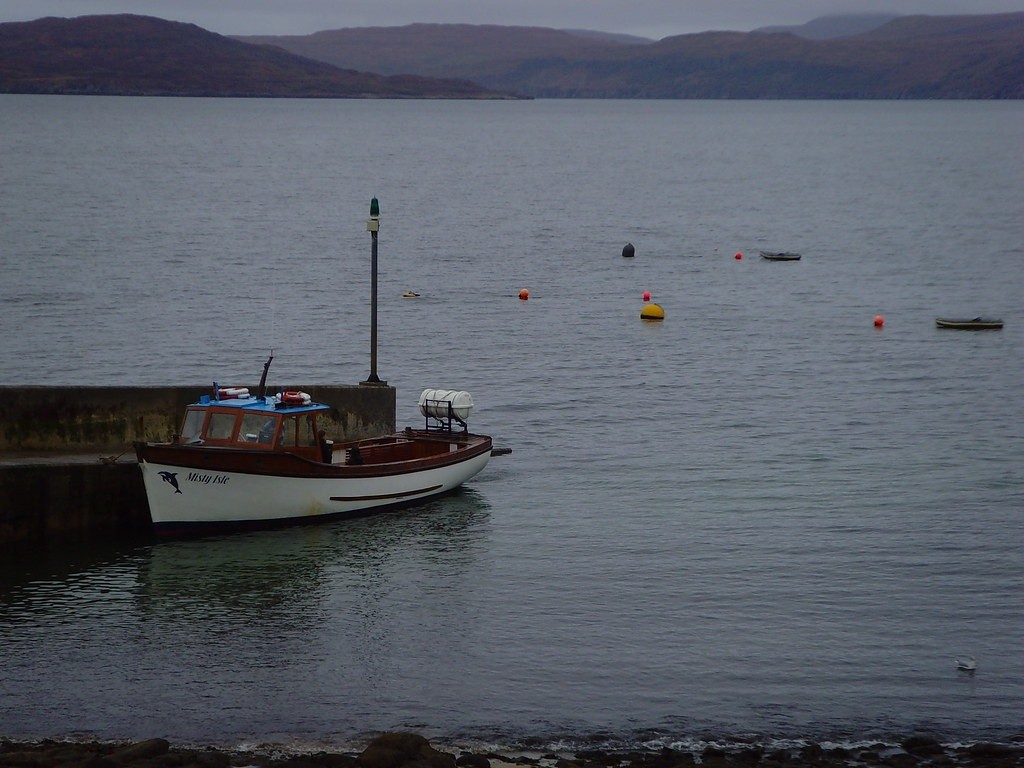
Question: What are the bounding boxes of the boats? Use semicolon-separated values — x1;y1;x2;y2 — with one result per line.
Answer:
128;197;514;537
933;316;1004;331
759;252;804;261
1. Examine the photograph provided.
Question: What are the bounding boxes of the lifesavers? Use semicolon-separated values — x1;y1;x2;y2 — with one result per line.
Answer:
276;398;311;406
212;387;249;395
276;392;311;401
215;393;250;400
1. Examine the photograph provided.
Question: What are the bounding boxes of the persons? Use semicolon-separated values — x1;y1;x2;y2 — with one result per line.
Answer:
311;430;333;464
